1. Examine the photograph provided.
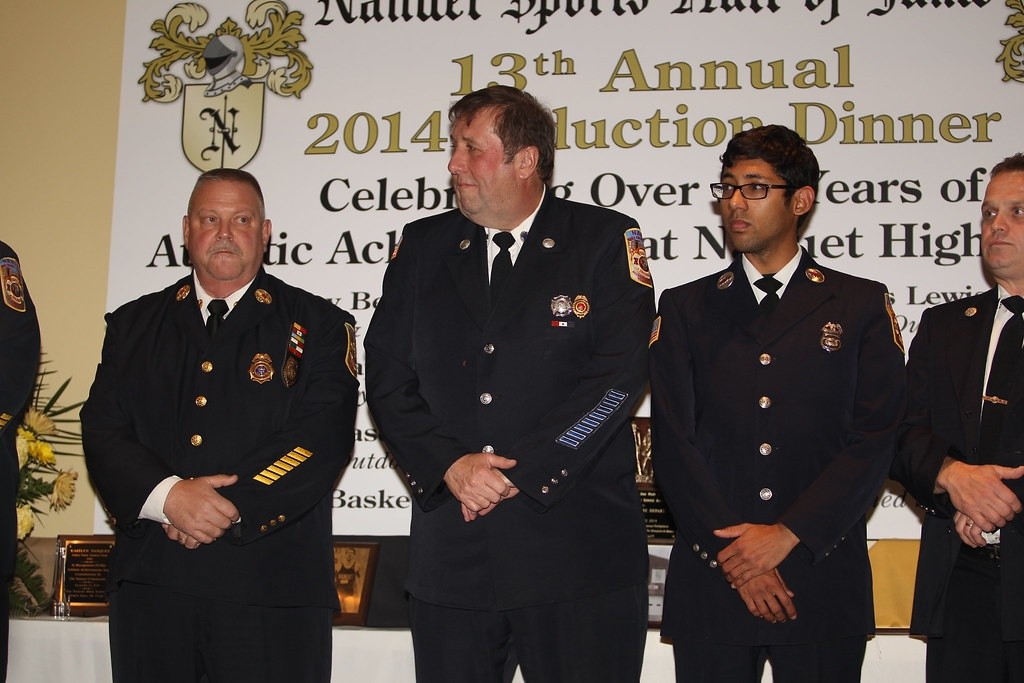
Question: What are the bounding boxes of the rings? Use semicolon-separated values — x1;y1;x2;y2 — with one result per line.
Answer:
966;523;972;527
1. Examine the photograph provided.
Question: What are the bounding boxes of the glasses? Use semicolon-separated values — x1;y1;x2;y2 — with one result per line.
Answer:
708;181;803;200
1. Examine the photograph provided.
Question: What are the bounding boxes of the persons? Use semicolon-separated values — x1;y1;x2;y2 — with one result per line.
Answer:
0;241;40;683
78;168;359;683
364;87;662;683
649;124;906;682
890;154;1024;683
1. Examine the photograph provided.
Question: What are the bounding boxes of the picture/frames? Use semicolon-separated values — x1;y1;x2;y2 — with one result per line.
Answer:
330;541;381;626
646;539;676;629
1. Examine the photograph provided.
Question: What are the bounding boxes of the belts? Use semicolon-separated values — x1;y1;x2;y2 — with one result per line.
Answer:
958;543;1003;567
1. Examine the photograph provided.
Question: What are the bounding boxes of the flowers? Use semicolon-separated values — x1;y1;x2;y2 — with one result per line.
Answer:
9;351;86;618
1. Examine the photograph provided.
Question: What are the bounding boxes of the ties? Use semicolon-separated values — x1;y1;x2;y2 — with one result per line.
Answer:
752;276;782;330
205;299;229;338
488;232;515;313
977;295;1024;465
